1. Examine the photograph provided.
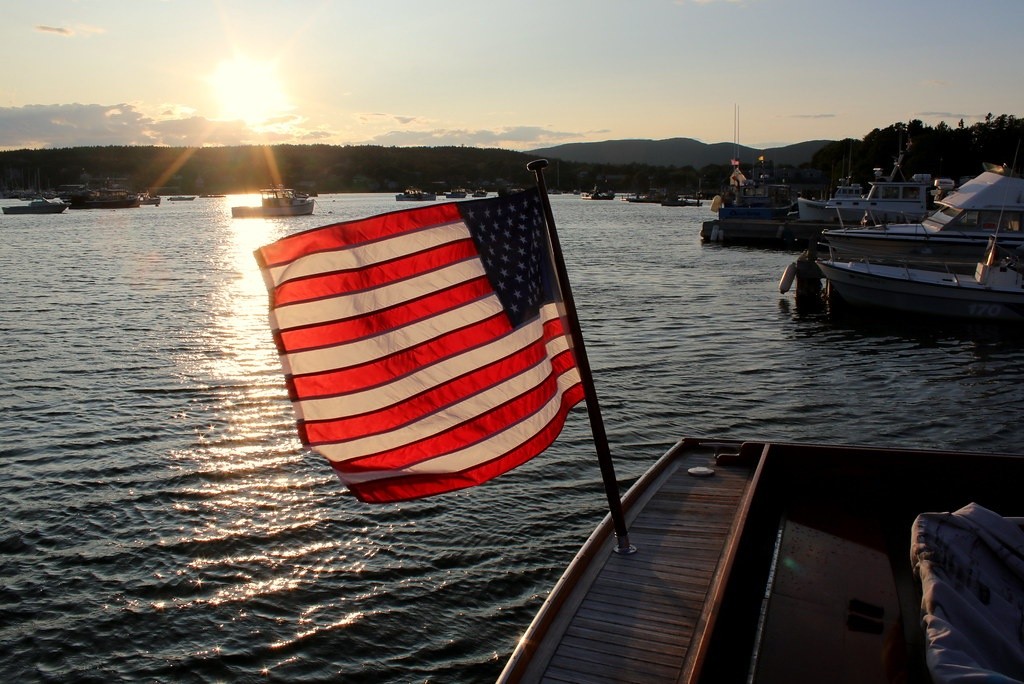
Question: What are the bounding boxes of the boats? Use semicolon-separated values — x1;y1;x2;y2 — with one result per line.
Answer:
716;156;794;219
170;196;196;201
308;189;318;197
662;201;688;206
472;190;488;197
45;194;54;199
813;244;1024;323
591;193;615;200
625;198;630;201
232;183;315;217
396;185;436;201
797;137;956;224
445;189;467;198
465;188;473;194
1;196;71;214
137;190;161;205
69;187;140;208
820;161;1024;264
493;435;1023;684
496;185;525;197
580;192;591;200
620;199;626;201
683;200;703;206
198;192;226;198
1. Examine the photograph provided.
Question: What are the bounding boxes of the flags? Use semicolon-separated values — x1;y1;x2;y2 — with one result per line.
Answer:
255;187;585;502
